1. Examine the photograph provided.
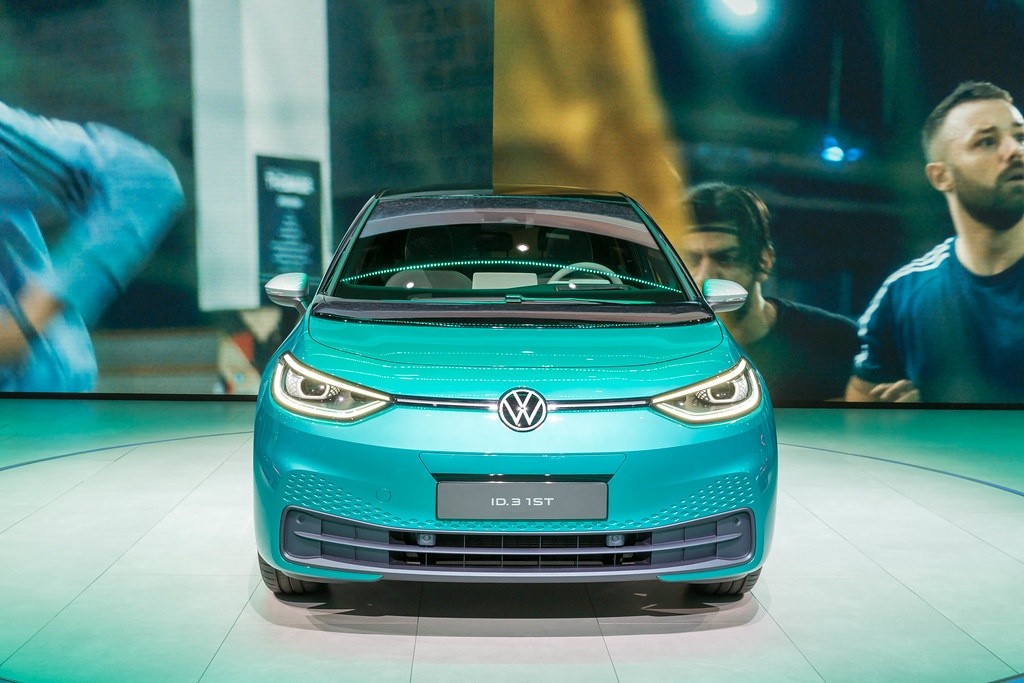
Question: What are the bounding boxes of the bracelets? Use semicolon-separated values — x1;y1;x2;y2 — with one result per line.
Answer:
6;294;38;342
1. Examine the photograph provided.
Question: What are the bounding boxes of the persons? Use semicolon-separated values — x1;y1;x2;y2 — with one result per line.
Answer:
0;103;183;392
677;181;860;405
844;80;1024;405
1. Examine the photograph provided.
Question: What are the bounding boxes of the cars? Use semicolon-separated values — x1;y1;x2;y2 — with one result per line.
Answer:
248;184;780;606
